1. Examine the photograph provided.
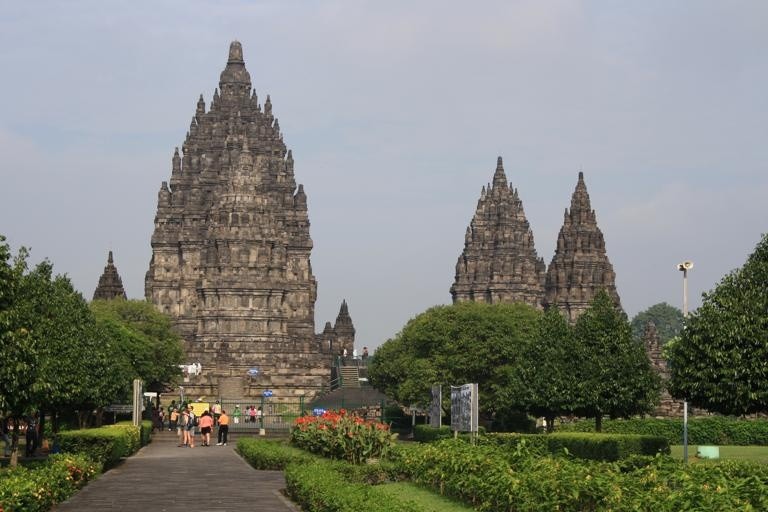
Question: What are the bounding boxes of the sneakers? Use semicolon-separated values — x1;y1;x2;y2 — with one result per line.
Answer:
216;442;223;446
201;443;210;447
178;443;195;448
224;443;227;446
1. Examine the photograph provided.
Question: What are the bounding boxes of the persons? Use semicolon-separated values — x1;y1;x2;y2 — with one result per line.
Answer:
343;346;347;358
363;347;368;353
1;407;11;458
23;409;38;458
142;395;265;448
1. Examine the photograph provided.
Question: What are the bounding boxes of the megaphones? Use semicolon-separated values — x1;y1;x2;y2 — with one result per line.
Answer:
682;260;693;270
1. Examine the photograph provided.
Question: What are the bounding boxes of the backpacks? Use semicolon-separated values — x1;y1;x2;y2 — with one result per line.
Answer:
191;412;199;426
186;414;193;427
